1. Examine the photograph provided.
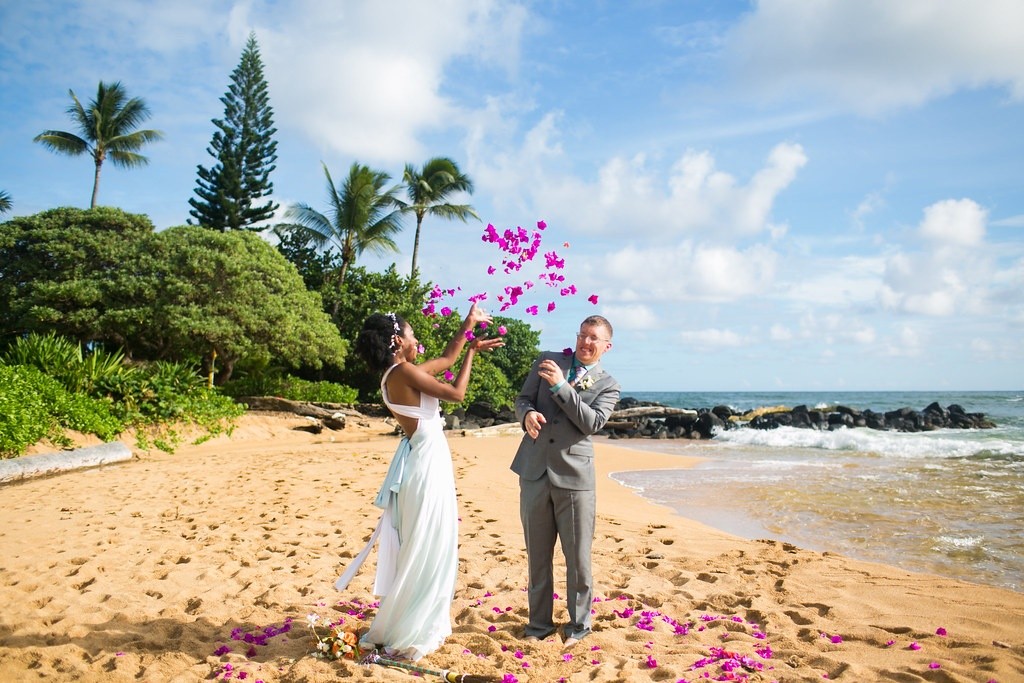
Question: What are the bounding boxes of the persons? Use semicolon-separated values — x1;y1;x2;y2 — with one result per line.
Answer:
509;315;622;651
359;298;506;663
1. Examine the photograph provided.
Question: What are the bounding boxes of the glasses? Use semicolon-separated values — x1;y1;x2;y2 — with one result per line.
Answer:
576;332;609;344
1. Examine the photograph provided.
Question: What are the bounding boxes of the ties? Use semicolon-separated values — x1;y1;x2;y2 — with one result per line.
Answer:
568;366;587;387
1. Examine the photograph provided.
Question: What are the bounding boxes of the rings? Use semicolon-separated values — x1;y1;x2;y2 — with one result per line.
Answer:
547;371;550;375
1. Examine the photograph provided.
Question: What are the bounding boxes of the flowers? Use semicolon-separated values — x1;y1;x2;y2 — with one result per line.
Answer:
576;374;595;391
306;613;365;662
